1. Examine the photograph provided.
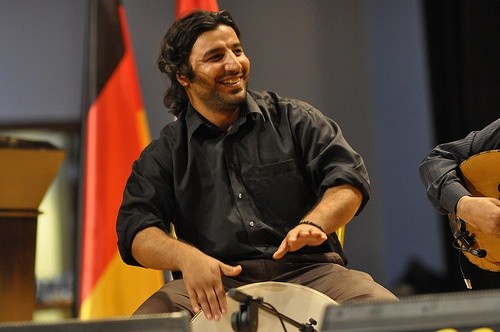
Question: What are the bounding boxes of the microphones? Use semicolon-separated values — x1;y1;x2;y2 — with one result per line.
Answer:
452;239;487;258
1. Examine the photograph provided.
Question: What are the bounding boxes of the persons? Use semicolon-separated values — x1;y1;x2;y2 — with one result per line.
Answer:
114;10;402;323
417;118;500;243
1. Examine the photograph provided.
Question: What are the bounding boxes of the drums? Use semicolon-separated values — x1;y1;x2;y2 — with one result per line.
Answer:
190;280;340;332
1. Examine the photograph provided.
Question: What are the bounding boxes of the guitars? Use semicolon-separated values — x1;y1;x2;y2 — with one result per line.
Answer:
446;149;500;274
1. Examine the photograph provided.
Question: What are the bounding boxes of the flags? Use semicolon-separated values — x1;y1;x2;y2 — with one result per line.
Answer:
75;1;164;323
175;0;221;23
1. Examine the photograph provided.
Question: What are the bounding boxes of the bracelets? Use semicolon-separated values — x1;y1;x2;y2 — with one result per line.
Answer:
295;221;331;241
